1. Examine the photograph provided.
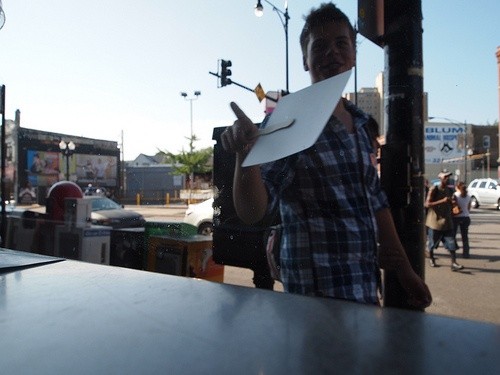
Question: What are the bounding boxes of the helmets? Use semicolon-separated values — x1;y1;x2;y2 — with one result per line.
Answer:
45;181;83;220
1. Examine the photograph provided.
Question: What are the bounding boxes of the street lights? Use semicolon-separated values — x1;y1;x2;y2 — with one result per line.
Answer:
58;139;76;182
254;0;291;96
428;117;468;183
181;91;200;189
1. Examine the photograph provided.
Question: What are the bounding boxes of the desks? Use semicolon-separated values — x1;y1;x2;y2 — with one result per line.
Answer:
0;244;500;375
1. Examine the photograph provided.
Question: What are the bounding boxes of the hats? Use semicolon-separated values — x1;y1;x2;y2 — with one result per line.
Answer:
438;169;453;179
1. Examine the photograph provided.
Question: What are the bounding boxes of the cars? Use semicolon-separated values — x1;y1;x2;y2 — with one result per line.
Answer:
95;188;106;195
83;196;146;226
84;189;92;196
185;196;215;237
431;179;458;192
467;178;500;211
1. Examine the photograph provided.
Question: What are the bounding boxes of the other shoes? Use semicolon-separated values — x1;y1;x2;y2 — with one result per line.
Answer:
429;258;436;268
451;262;464;272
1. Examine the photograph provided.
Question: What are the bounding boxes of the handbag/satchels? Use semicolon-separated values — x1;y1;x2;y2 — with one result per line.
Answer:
263;225;282;283
426;208;446;231
452;205;462;216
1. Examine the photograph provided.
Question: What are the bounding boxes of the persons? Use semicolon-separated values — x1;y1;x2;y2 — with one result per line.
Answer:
29;153;56;173
76;156;115;180
96;188;102;196
453;180;474;258
424;169;464;271
85;184;93;196
222;2;433;309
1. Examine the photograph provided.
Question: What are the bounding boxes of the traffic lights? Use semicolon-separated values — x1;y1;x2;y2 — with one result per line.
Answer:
221;60;231;88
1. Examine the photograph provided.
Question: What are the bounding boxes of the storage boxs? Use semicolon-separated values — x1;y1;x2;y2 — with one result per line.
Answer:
142;221;200;271
